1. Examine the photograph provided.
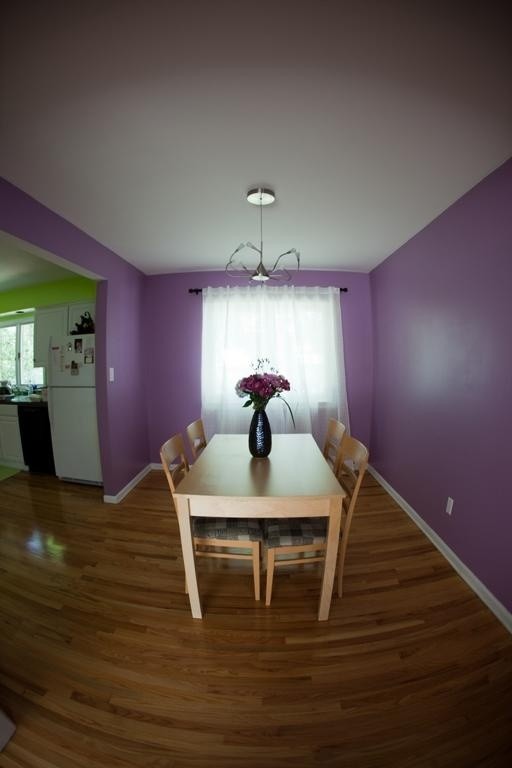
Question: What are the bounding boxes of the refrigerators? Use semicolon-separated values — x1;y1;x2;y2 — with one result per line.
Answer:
45;334;103;486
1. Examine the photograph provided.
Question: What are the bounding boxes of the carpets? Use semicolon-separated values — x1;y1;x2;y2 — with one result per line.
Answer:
0;467;20;481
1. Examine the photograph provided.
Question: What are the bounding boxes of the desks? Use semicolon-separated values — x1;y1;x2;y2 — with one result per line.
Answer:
174;432;348;627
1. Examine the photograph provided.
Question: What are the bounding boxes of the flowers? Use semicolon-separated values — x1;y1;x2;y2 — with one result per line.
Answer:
234;357;298;431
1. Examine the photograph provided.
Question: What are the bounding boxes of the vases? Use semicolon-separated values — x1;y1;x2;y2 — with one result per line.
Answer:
248;409;272;458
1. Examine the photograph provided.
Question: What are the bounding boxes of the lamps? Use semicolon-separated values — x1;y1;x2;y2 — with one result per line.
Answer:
226;187;303;286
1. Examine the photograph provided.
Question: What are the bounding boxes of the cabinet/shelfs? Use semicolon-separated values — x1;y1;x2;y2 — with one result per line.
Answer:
0;404;27;471
32;297;96;367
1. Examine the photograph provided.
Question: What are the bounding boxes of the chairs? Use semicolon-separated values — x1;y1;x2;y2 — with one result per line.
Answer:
263;434;369;607
323;416;348;466
186;417;207;465
159;432;265;602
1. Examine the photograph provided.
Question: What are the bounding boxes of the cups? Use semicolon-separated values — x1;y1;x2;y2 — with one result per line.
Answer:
27;386;33;396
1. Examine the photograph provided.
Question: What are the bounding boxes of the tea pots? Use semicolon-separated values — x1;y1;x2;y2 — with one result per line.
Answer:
0;379;12;395
